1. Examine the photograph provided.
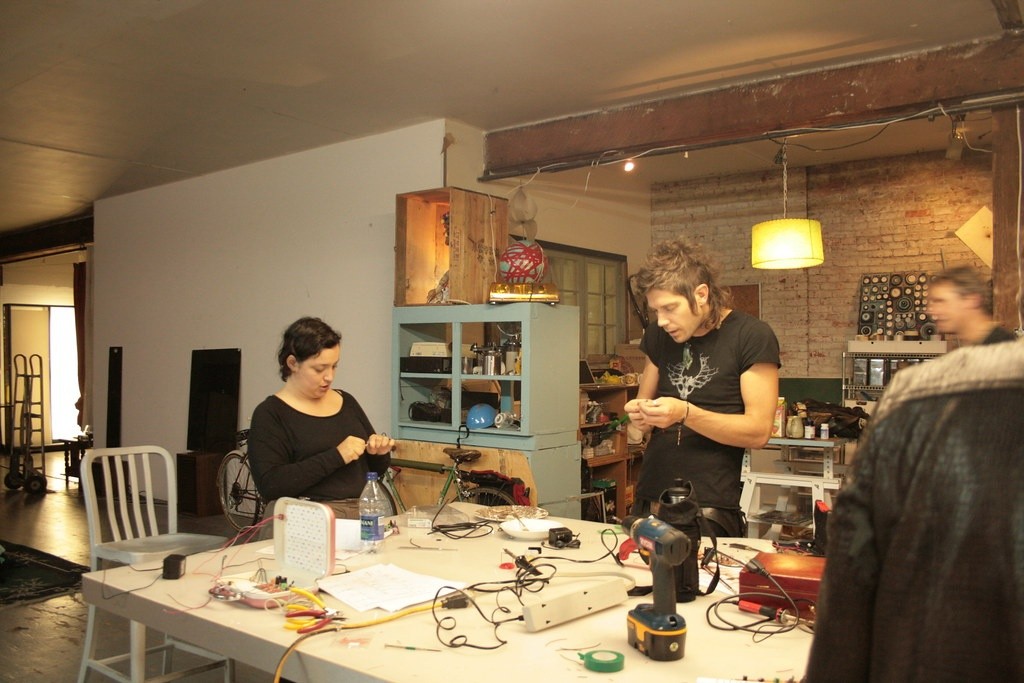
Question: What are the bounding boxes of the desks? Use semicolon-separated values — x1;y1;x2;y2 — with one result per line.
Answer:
58;440;92;492
81;501;813;683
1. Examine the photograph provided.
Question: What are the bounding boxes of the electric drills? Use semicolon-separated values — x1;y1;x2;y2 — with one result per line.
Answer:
612;514;691;662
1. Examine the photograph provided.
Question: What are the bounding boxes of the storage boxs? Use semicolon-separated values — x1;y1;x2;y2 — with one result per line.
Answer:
592;479;615;488
739;552;824;618
400;357;452;373
625;482;634;507
409;340;477;357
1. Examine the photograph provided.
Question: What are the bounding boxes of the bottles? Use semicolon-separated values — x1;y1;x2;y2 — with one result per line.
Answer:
485;351;497;375
804;421;815;439
820;423;829;440
505;336;517;375
358;472;385;551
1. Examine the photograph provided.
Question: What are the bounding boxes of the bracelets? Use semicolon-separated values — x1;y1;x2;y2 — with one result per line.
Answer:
678;401;689;445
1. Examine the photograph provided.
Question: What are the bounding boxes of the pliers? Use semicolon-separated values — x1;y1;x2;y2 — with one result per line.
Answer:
284;587;348;633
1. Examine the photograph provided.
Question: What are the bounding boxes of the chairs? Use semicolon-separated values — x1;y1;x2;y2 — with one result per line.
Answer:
78;446;236;683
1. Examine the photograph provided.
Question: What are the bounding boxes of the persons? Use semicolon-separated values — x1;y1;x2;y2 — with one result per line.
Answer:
249;317;396;541
805;338;1024;683
926;265;1015;346
623;238;783;537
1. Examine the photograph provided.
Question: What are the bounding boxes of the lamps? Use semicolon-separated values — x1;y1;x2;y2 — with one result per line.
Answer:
752;135;824;269
946;111;963;160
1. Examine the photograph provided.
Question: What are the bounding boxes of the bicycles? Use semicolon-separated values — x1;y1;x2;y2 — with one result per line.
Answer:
216;429;268;533
378;444;529;516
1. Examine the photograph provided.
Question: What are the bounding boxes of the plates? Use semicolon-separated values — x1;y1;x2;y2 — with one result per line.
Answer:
476;505;548;522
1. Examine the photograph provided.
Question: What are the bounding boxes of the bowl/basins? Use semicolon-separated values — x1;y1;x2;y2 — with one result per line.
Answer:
499;520;562;539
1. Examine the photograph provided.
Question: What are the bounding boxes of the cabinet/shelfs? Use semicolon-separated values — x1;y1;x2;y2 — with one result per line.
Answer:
842;352;945;415
176;452;227;517
390;186;645;525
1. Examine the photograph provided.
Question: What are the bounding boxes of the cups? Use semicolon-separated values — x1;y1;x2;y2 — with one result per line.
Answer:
786;416;803;438
429;389;450;408
462;357;473;373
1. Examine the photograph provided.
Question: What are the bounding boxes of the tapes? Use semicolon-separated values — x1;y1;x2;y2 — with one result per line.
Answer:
579;650;625;673
